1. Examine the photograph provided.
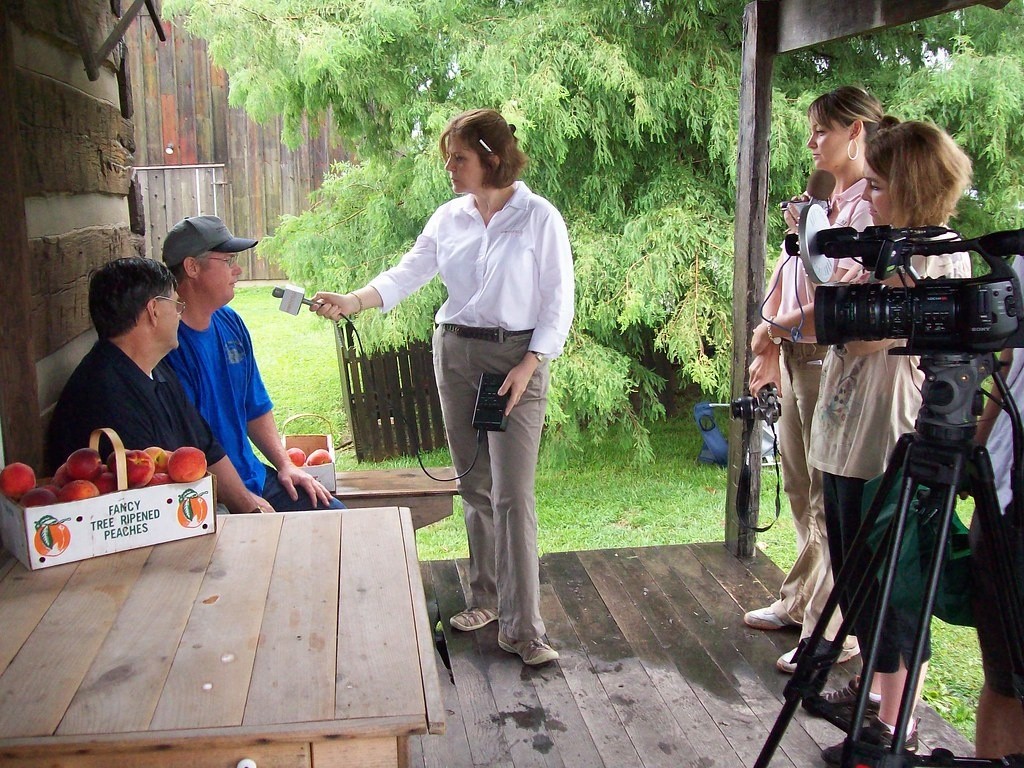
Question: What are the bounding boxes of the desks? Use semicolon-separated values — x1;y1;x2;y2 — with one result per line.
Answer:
0;506;447;768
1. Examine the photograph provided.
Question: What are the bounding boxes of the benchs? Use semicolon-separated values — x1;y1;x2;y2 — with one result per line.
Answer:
331;467;461;532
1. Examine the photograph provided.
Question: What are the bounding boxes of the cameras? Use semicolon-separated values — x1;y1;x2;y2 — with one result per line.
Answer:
732;383;782;425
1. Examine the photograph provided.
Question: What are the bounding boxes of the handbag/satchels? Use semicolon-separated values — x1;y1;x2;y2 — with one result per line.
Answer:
859;464;976;630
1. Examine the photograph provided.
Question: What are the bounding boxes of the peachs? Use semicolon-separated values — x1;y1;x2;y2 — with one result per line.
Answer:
286;447;333;467
0;446;207;507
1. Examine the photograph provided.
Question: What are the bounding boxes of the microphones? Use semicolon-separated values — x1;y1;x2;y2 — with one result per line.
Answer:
272;283;324;316
804;169;836;203
819;226;947;258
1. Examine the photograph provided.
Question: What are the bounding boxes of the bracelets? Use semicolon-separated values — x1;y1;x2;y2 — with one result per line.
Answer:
252;506;264;513
351;292;363;315
767;316;782;345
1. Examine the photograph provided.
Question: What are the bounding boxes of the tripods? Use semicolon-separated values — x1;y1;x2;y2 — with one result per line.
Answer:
753;346;1024;768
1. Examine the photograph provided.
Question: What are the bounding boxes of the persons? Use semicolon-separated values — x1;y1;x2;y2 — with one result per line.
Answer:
961;252;1024;768
47;257;266;514
309;109;575;664
803;121;976;768
743;85;885;672
164;214;348;514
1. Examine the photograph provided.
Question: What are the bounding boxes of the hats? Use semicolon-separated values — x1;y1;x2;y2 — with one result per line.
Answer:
162;214;259;268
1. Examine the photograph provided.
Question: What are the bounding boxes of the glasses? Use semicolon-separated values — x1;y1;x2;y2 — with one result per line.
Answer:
199;254;239;267
146;296;186;315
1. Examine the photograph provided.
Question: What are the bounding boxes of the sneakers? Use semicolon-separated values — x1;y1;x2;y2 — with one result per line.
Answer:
497;631;559;664
449;606;498;631
776;644;860;672
744;606;788;629
803;675;881;718
821;714;921;767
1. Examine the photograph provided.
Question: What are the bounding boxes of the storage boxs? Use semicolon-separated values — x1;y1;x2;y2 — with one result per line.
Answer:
0;470;217;571
281;433;337;494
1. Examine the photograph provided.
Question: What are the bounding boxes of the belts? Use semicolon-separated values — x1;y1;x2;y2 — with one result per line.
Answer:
444;324;535;342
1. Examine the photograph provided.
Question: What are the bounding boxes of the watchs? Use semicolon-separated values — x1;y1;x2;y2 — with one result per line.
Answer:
534;351;544;362
